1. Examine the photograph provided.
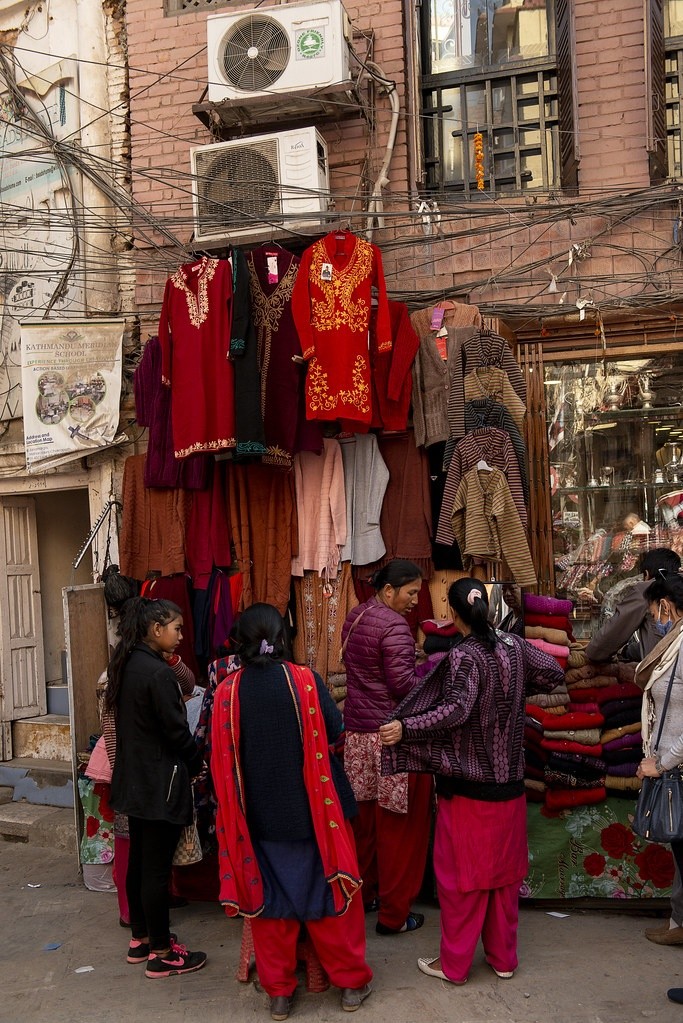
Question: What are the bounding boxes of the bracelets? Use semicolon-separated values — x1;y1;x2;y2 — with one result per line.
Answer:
656;758;663;775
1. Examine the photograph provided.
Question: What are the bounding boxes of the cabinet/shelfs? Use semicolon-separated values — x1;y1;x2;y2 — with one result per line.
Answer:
547;405;683;609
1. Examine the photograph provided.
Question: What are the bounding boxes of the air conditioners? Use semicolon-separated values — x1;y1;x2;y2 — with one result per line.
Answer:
207;1;356;123
191;125;331;241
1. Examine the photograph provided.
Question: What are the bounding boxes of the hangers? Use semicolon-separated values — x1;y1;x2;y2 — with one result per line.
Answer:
183;217;495;472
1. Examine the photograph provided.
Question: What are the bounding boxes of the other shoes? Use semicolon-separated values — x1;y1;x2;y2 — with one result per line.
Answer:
418;957;468;984
364;897;380;912
645;924;683;944
341;982;371;1011
169;894;190;909
667;988;683;1004
485;956;514;979
376;911;424;935
270;990;297;1020
119;917;172;927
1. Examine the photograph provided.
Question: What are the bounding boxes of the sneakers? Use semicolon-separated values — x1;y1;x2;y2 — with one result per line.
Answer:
127;933;177;963
145;937;207;978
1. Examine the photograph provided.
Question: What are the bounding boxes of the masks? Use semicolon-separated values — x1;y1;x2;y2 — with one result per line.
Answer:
656;605;672;637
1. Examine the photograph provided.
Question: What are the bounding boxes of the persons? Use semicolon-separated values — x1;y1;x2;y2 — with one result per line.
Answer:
96;558;566;1020
586;547;683;1003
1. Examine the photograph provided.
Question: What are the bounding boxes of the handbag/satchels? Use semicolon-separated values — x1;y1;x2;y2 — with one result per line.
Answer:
631;772;683;843
172;783;203;865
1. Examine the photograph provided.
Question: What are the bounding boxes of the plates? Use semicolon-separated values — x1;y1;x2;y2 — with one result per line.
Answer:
619;480;643;485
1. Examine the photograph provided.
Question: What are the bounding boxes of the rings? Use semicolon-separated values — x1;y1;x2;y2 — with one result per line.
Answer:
638;766;640;769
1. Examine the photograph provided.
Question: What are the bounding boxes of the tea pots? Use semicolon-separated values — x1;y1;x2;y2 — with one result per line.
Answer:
547;374;656;410
